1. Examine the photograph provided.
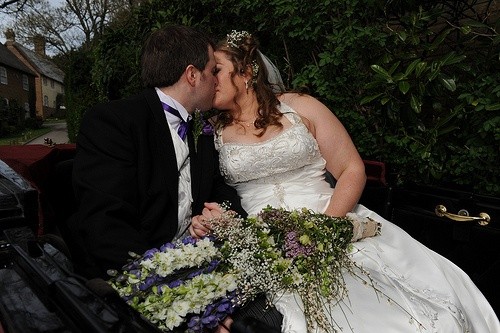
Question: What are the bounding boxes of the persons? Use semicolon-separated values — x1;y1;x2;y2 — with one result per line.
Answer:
188;30;500;333
72;25;283;332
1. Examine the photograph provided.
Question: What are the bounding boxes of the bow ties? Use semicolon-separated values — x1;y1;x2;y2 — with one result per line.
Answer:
161;101;193;141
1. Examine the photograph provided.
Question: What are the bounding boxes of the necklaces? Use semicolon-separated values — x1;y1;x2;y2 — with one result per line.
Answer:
233;119;260;129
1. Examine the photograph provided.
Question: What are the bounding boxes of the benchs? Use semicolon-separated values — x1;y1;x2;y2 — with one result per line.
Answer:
0;144;389;333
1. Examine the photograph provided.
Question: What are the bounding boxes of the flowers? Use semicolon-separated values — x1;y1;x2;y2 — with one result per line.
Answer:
191;107;213;152
250;60;259;86
225;29;251;49
106;200;428;333
